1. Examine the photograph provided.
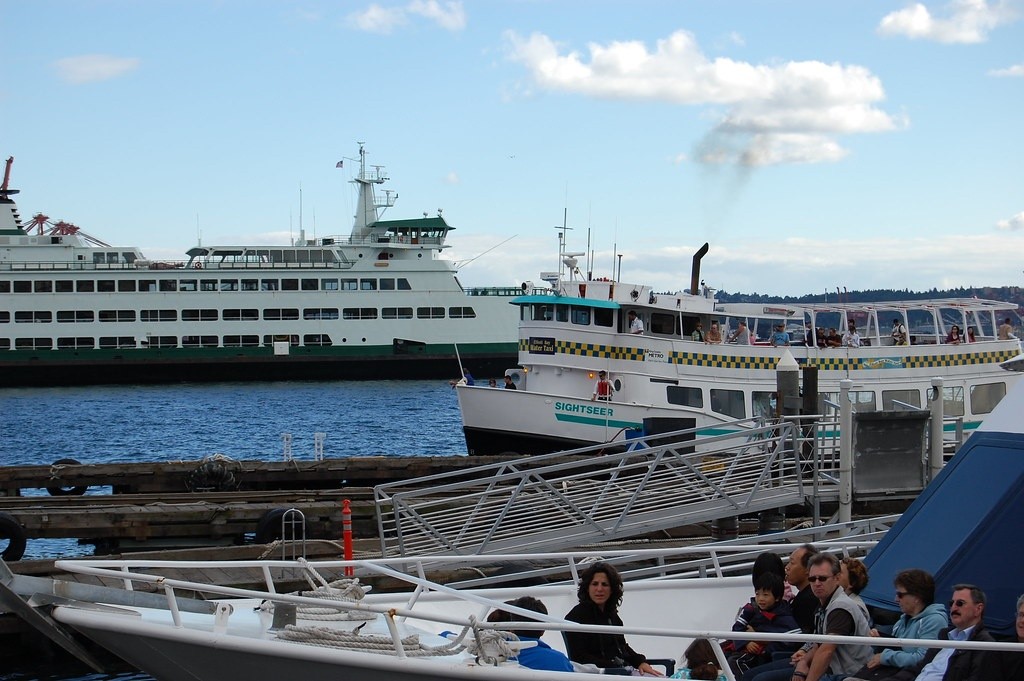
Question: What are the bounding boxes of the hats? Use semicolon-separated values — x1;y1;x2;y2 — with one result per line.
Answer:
849;326;855;330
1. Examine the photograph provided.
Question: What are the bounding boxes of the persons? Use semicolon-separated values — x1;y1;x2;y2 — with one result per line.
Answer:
719;543;875;681
691;322;706;341
842;568;950;680
963;326;976;343
671;637;730;680
562;560;667;677
978;592;1024;681
772;322;789;347
891;318;907;346
999;317;1019;340
627;310;645;335
705;324;722;344
591;370;616;402
891;582;1005;681
503;375;518;389
946;325;961;345
805;318;860;347
449;367;475;384
728;321;755;345
488;596;575;671
489;378;497;388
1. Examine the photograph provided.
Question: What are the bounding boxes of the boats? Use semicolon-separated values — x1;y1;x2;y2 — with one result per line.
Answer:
0;139;617;380
456;202;1023;469
0;366;1024;681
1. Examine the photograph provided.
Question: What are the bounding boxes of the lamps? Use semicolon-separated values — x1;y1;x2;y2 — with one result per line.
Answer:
589;373;593;379
524;367;527;373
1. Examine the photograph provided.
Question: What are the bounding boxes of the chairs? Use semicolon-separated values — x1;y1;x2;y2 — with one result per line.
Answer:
560;630;676;677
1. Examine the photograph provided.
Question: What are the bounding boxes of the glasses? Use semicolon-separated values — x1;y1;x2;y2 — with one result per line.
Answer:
953;328;956;330
899;337;902;338
697;325;702;327
808;576;833;582
711;327;715;329
1015;612;1024;619
896;591;909;599
490;382;495;384
818;332;823;335
949;600;966;607
848;322;850;324
599;375;603;377
778;325;783;328
